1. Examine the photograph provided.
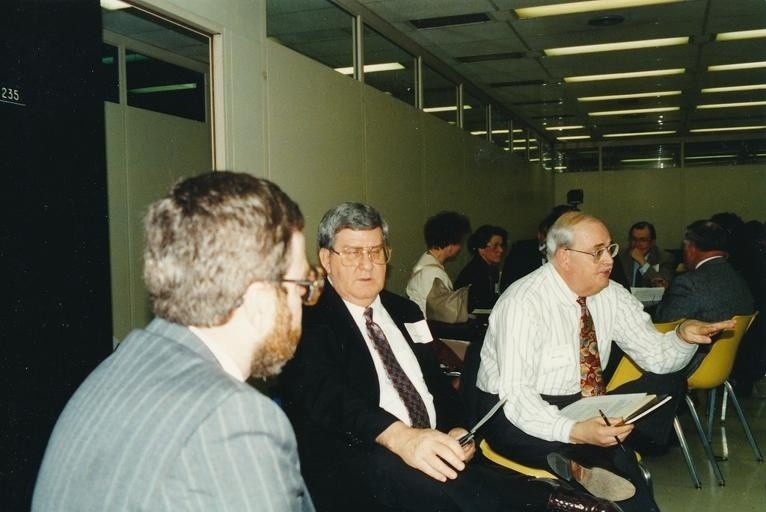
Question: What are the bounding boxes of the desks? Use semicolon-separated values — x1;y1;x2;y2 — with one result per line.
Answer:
623;285;667;314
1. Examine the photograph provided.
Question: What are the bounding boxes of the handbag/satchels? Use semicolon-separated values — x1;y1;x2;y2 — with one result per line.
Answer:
424;277;472;325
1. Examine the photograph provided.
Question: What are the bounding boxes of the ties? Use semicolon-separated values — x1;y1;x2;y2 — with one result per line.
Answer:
363;311;436;429
575;296;610;398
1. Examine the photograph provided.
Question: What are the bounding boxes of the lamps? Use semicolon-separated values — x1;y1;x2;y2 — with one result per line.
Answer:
672;305;760;487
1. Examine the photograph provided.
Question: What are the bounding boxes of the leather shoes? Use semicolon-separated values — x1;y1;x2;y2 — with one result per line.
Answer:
526;477;626;512
546;448;638;501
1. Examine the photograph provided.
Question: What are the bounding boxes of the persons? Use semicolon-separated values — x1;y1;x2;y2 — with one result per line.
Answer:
405;210;470;322
31;170;324;512
475;211;737;511
653;221;754;325
452;224;507;311
295;203;480;512
618;222;674;288
499;206;631;293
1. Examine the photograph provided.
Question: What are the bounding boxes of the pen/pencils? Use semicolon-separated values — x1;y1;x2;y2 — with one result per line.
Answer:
599;408;627;452
457;429;477;449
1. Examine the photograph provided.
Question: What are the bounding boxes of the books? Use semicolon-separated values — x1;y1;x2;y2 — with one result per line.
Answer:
558;392;672;426
453;394;508;446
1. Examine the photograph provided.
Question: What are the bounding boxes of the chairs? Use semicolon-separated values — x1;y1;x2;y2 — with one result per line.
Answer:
604;318;700;489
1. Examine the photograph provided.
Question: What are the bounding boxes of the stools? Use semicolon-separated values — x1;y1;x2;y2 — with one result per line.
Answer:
477;430;656;511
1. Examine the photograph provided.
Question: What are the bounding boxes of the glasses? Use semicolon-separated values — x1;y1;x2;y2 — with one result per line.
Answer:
326;244;393;267
274;275;318;305
566;244;620;264
